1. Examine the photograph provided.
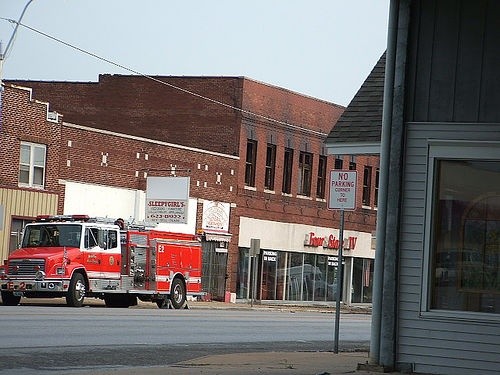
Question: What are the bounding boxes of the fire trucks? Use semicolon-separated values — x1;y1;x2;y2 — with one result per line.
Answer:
0;215;207;309
